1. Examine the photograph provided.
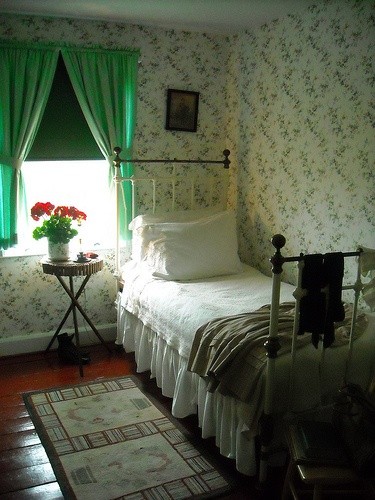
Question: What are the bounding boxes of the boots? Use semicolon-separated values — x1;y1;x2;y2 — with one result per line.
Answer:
58;334;91;366
58;333;90;358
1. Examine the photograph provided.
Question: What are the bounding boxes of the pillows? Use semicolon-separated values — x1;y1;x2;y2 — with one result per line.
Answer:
136;209;241;281
128;209;216;263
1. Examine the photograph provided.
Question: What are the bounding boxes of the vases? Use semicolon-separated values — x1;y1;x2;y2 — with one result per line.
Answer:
48;238;69;259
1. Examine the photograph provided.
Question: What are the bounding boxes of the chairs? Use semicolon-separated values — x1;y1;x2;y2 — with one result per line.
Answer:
282;424;360;500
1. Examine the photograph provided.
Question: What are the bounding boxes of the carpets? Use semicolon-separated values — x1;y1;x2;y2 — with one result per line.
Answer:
23;374;233;500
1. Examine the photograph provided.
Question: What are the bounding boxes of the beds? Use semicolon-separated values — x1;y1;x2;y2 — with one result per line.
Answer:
113;146;375;500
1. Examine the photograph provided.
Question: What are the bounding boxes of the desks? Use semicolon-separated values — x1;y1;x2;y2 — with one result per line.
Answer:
43;258;112;377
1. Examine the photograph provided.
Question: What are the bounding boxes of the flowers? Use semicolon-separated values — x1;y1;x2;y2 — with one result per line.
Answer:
30;202;87;244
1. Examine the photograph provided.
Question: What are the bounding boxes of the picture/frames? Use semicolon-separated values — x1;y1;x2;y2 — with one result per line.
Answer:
165;89;200;132
